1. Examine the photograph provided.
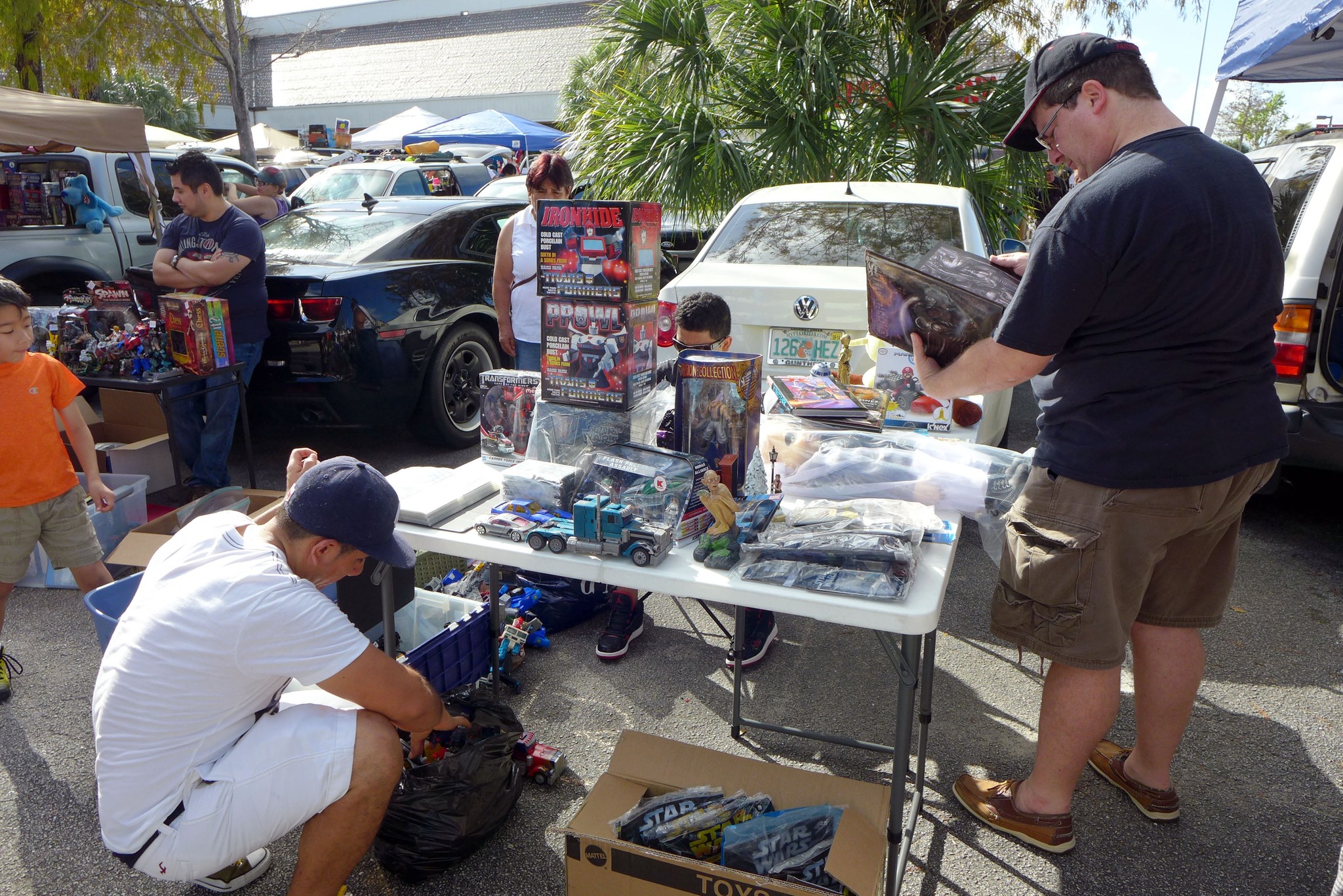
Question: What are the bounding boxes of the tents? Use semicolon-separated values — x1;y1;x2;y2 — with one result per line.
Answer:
214;106;573;176
1204;0;1343;140
0;84;166;254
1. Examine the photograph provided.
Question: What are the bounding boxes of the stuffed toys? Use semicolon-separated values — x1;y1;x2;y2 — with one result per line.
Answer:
61;174;124;234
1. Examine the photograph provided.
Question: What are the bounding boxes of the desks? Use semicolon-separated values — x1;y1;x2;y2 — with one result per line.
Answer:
64;359;258;491
371;388;988;896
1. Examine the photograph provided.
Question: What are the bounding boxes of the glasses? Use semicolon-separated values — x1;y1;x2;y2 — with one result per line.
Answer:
255;178;277;185
672;333;726;352
1035;88;1082;150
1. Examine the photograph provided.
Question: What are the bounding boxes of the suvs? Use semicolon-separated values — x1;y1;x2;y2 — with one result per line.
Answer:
248;145;724;271
1241;128;1342;504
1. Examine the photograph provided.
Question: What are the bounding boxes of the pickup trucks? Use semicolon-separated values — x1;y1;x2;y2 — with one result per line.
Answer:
0;148;269;311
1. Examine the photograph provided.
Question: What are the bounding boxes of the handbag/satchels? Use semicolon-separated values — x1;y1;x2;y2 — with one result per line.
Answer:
516;569;607;636
373;682;528;886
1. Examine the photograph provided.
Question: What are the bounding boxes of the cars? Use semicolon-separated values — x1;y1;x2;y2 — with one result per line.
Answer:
243;192;539;450
653;180;1033;450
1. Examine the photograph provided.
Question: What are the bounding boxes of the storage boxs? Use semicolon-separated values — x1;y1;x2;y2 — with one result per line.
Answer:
57;387;177;495
537;296;660;411
103;488;289;572
549;729;892;896
674;346;764;497
478;368;541;467
81;568;146;658
362;584;493;697
534;198;663;303
157;290;235;377
11;470;152;591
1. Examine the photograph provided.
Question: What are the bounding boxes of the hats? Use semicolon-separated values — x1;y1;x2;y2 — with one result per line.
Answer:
253;165;285;185
284;456;417;570
1003;33;1142;152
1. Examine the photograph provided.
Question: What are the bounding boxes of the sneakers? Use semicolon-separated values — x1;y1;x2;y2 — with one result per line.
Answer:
726;607;778;667
190;846;271;893
595;591;644;659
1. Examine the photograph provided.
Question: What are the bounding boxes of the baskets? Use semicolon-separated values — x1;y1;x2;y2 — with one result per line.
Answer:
333;587;491;696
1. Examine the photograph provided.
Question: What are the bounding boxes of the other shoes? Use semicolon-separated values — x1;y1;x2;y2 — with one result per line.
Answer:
1087;738;1181;824
192;484;220;501
952;773;1076;855
0;643;12;700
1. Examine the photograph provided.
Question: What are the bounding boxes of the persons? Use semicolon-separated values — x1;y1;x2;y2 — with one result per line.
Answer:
151;145;271;502
593;293;778;668
492;152;576;370
422;169;446;178
220;166;288;229
487;163;517;180
906;36;1292;856
91;455;474;896
351;299;382;391
479;219;924;537
1;274;119;698
33;311;177;375
